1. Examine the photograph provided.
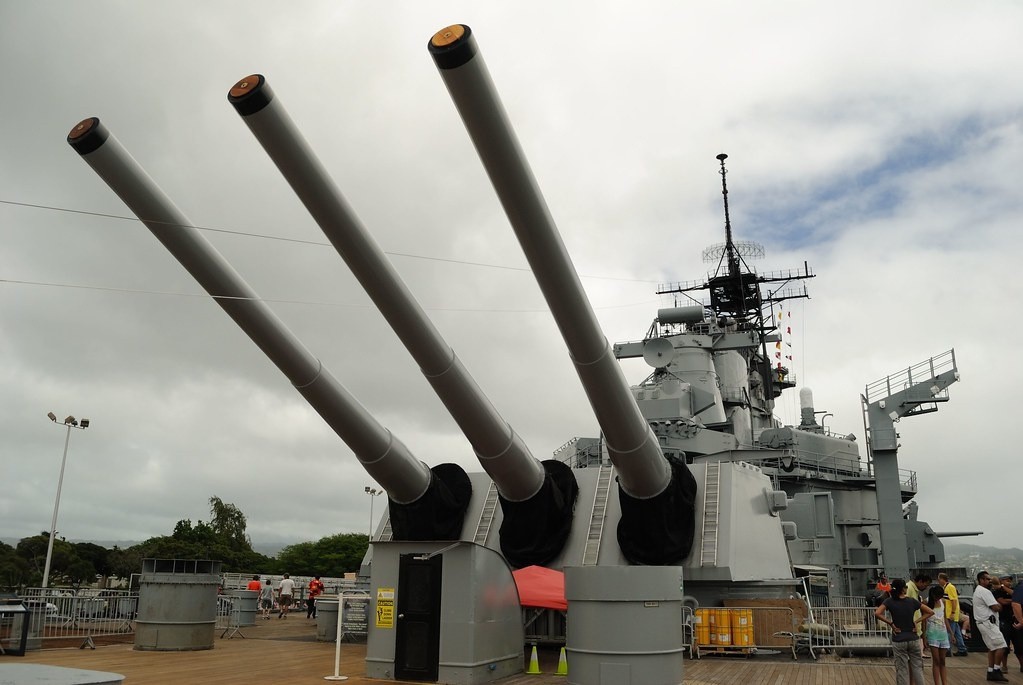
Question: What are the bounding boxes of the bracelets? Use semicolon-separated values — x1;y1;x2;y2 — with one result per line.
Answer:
889;622;894;626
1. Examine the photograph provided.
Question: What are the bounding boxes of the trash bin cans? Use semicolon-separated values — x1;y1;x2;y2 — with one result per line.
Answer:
9;599;47;650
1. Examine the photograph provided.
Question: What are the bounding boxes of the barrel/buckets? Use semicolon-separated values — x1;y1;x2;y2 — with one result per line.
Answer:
694;608;754;646
233;589;259;627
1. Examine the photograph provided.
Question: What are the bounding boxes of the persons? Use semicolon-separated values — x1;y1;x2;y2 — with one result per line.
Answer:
305;574;325;619
991;575;1023;674
875;578;935;685
959;609;971;639
1012;580;1023;629
245;575;262;598
277;572;295;619
903;573;933;661
259;580;277;621
937;573;968;657
972;570;1011;682
920;586;957;685
875;575;893;594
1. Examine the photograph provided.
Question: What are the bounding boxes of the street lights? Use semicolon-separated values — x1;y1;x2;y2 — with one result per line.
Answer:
40;411;89;608
365;487;383;547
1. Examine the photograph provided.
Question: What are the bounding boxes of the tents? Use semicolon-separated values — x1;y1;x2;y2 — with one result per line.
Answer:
509;564;567;611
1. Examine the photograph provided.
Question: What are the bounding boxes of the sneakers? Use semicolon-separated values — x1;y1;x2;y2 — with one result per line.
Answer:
1000;667;1007;673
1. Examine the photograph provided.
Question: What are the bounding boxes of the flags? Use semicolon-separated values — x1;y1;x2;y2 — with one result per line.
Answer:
775;303;794;369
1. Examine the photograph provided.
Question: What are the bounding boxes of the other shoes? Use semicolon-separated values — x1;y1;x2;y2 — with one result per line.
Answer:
278;612;287;618
986;671;993;682
306;612;316;619
261;615;271;620
993;668;1008;681
